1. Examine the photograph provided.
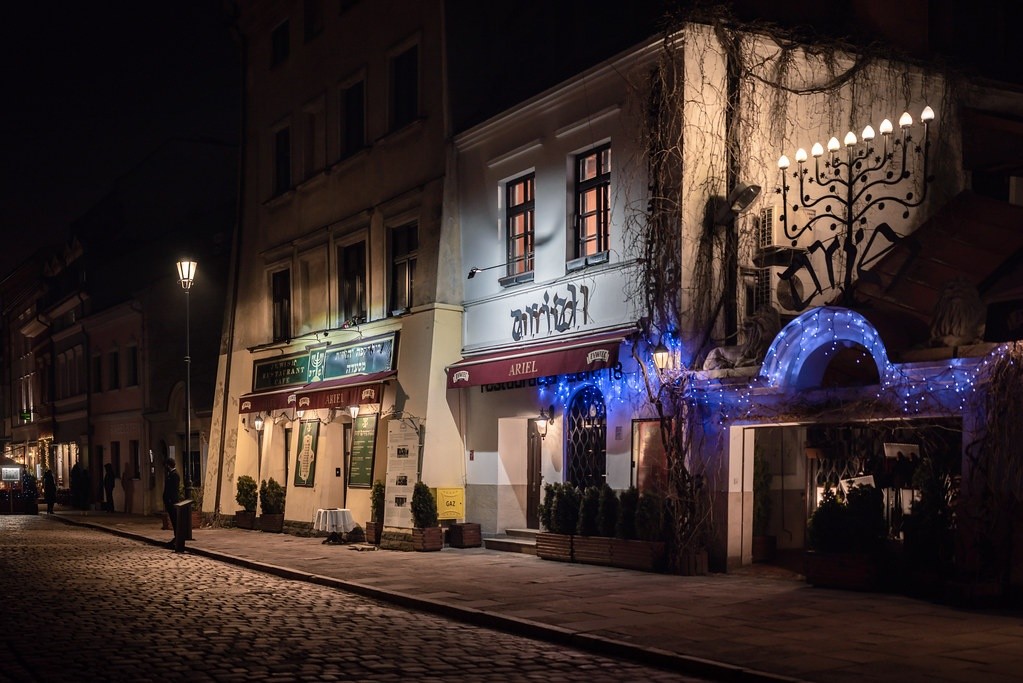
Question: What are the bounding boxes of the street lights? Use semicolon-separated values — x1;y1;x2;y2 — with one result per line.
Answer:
176;252;197;539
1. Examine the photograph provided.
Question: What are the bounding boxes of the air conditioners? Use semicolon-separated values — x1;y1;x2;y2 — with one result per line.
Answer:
756;265;833;317
759;206;816;248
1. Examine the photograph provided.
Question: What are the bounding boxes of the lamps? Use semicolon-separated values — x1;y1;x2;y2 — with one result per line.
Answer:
324;325;359;337
250;347;284;354
254;411;263;431
297;410;305;418
468;255;533;279
285;335;318;344
349;405;360;419
778;103;936;306
716;180;763;225
533;404;554;440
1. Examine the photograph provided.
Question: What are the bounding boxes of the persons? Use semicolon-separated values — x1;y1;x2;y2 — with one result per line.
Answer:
104;463;115;513
164;458;180;544
44;470;56;514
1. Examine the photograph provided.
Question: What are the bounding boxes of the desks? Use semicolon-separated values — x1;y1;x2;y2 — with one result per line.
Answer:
314;509;355;544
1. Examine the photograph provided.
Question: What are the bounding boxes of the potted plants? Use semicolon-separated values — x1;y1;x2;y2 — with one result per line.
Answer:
236;475;257;529
536;480;676;571
411;483;443;551
752;435;776;564
804;484;889;589
365;480;385;543
161;475;201;529
260;477;286;532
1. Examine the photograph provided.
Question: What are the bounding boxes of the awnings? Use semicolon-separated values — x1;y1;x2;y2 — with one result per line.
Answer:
296;369;398;410
239;383;309;414
448;328;638;389
